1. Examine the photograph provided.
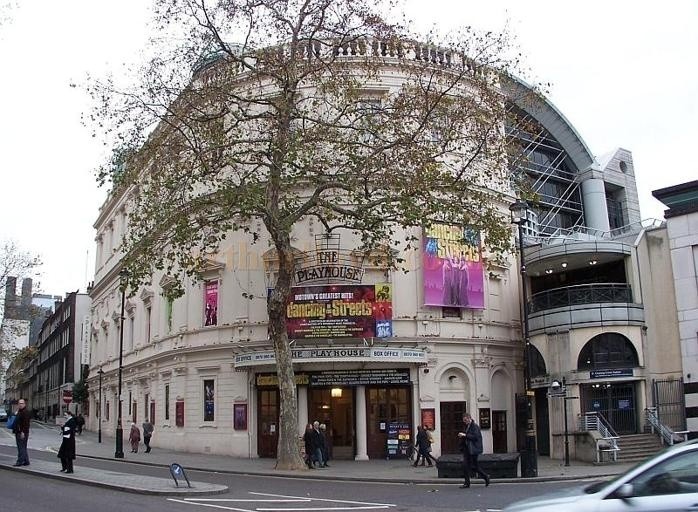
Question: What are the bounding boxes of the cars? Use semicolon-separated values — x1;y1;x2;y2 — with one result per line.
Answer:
500;437;697;512
0;406;8;423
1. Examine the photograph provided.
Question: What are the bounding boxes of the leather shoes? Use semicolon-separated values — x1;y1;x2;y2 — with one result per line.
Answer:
411;463;433;467
309;463;329;469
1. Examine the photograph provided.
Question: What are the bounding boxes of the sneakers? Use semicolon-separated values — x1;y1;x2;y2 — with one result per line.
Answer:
13;460;30;466
485;474;490;486
459;484;470;488
144;447;151;453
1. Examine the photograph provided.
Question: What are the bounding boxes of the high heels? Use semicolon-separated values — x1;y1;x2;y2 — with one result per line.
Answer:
61;465;73;474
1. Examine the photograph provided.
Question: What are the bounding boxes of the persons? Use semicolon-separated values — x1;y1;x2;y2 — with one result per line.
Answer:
457;412;491;488
56;410;85;474
128;422;141;453
412;424;438;467
205;296;217;326
443;252;469;306
12;398;31;466
142;418;154;453
301;420;331;469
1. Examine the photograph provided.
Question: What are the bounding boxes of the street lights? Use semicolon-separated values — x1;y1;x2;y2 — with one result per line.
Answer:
95;361;104;444
113;266;130;459
508;194;538;478
552;376;571;467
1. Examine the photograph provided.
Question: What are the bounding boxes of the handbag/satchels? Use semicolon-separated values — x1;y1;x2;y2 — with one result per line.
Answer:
6;415;17;429
457;436;465;450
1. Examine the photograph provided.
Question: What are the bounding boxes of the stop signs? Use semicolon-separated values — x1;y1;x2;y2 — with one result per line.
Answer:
62;392;72;404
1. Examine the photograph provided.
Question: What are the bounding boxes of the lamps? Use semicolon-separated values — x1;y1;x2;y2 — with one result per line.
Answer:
330;387;343;397
